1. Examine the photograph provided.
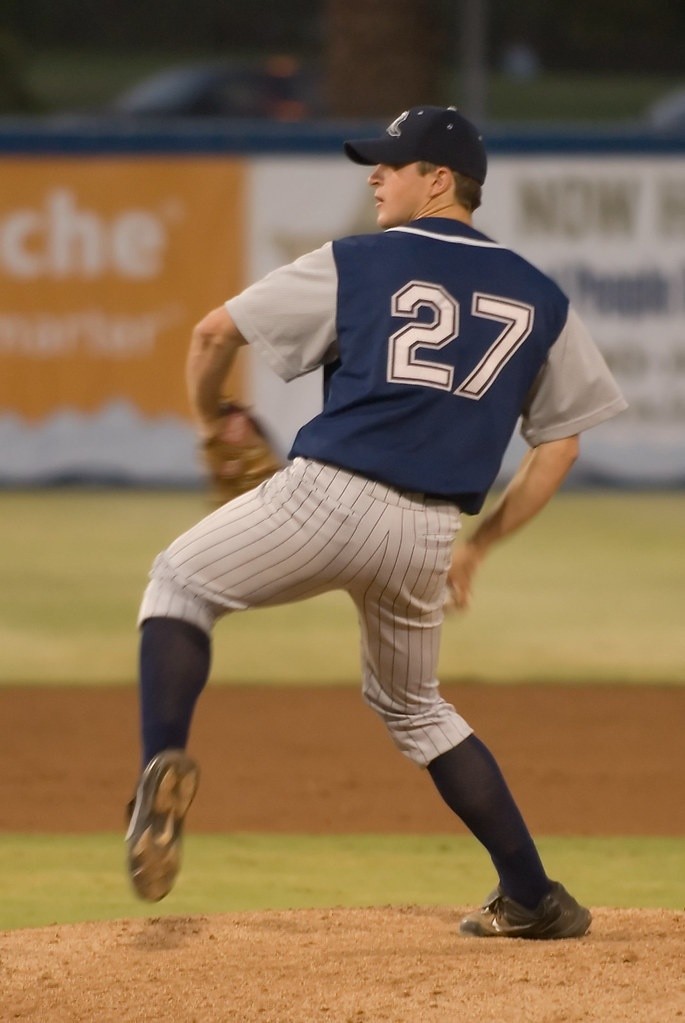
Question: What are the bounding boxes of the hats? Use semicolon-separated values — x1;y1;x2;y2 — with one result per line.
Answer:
342;106;488;185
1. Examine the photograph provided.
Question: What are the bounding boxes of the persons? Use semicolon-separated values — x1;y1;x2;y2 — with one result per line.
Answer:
124;106;631;940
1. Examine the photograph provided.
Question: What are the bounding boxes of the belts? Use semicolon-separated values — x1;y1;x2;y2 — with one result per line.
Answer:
358;469;457;504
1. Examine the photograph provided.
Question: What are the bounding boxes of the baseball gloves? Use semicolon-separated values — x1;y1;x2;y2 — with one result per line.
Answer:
199;403;285;507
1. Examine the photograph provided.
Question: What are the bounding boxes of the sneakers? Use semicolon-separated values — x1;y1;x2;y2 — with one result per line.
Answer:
124;748;199;904
458;880;591;937
